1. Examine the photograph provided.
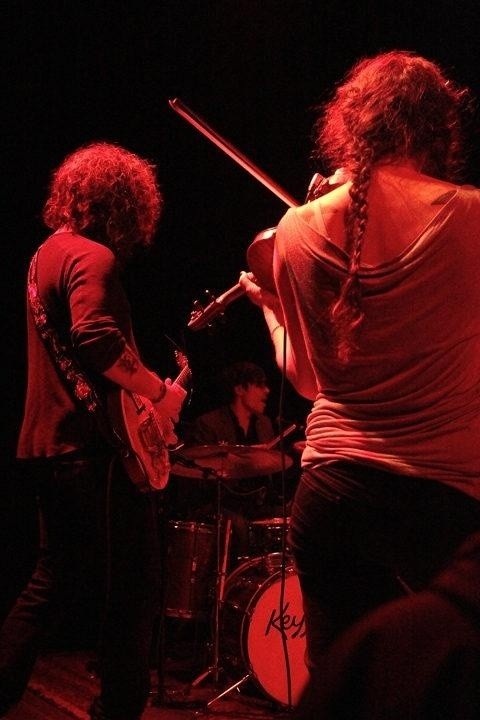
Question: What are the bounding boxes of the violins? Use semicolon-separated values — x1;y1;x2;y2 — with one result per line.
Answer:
187;168;357;335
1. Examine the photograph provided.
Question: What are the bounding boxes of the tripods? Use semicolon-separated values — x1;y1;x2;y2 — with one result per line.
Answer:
174;477;237;702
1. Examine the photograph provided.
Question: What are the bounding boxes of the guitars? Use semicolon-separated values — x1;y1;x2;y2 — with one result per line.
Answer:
102;349;193;495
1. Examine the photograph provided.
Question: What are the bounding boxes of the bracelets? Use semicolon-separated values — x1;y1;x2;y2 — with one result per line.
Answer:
423;580;480;630
152;385;168;404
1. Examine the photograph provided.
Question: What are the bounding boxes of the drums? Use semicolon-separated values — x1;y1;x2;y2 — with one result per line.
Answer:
208;553;309;709
160;519;223;623
245;516;295;555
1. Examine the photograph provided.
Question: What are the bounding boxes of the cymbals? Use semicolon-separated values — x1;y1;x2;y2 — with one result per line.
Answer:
294;440;306;450
171;444;292;480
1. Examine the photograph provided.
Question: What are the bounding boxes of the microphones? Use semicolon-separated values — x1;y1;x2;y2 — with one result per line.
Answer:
270;412;305;432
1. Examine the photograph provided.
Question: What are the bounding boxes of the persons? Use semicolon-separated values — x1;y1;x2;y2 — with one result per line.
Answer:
316;528;479;720
166;360;309;567
0;140;192;720
241;46;480;720
